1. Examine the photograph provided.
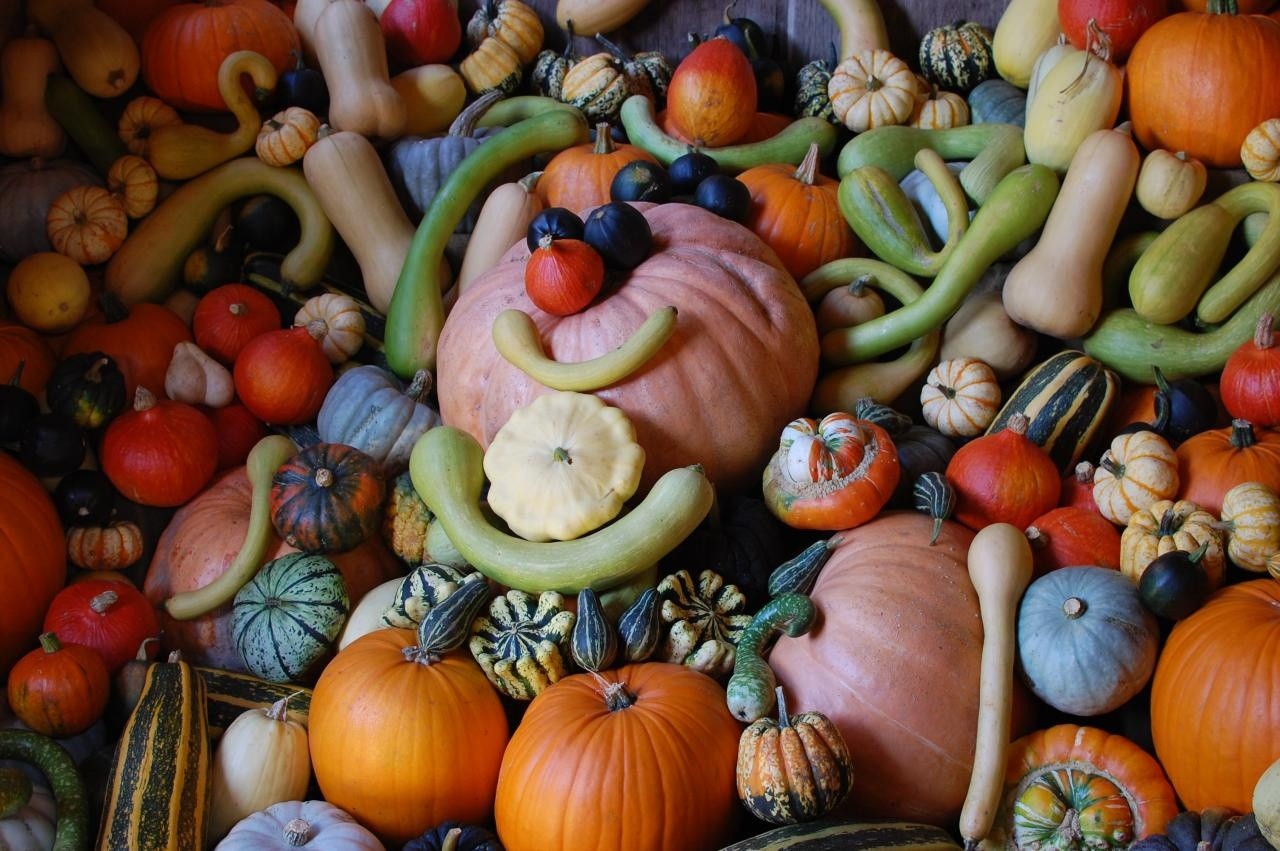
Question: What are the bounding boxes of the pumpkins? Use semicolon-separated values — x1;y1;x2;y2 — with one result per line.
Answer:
0;0;1280;851
